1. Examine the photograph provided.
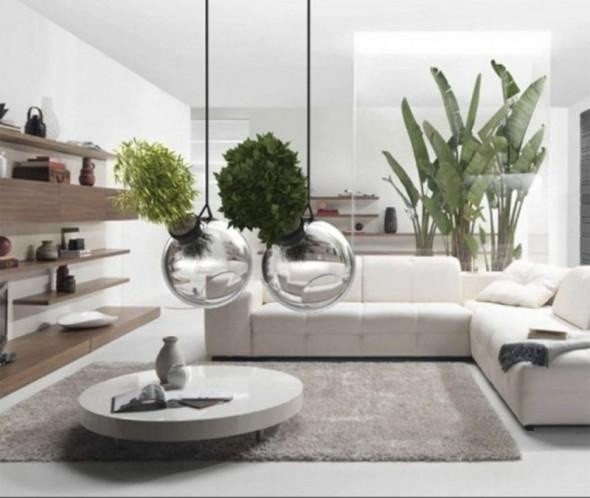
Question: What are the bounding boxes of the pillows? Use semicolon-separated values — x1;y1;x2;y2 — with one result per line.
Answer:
473;258;569;307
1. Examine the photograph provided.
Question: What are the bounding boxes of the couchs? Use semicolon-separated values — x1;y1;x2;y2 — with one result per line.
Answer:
204;255;590;430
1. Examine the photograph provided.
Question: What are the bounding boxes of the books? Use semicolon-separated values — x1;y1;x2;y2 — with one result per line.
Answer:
109;382;235;416
526;327;569;341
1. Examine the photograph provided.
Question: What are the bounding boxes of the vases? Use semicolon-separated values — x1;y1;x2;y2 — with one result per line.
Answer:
79;157;96;187
154;335;191;388
55;265;76;294
383;205;397;234
35;241;59;261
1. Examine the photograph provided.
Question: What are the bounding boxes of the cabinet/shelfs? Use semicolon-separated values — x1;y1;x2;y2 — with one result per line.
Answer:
0;126;163;398
305;196;501;237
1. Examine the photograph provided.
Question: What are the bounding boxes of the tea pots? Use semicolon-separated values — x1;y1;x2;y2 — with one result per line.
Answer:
23;104;46;135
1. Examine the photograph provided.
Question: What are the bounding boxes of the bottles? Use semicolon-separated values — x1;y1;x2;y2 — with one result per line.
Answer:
155;336;189;388
0;150;9;178
384;206;397;231
56;263;76;292
34;239;59;260
77;155;97;186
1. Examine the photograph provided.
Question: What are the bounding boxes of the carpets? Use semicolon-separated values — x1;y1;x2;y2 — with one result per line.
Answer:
0;358;521;466
